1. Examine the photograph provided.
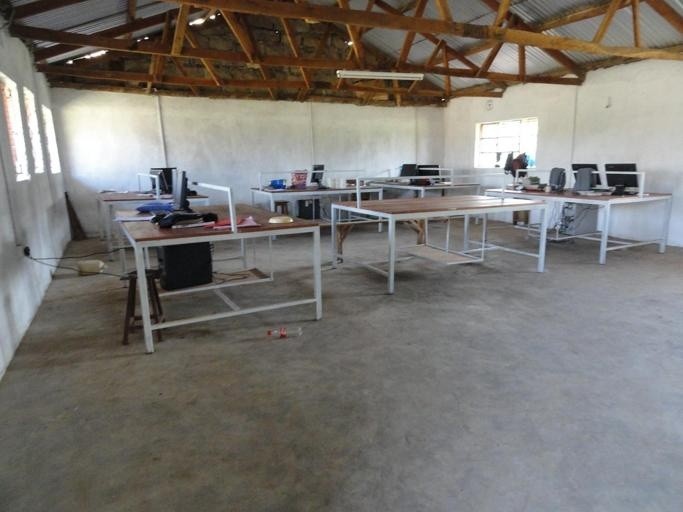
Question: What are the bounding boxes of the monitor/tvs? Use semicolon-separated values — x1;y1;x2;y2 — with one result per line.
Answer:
571;164;609;194
604;163;639;196
400;163;416;184
418;164;440;183
311;165;329;189
150;169;168;195
151;167;177;194
174;171;192;212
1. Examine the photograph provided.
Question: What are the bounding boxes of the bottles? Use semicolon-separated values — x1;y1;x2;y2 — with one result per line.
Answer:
268;327;302;338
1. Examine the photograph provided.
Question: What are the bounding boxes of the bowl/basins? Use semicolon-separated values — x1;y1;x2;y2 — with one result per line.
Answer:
268;216;293;223
271;180;287;189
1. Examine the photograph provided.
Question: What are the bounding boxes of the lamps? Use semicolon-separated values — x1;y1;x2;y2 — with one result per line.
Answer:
337;67;425;85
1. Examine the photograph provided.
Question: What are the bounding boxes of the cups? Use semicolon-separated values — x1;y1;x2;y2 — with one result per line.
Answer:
544;185;552;193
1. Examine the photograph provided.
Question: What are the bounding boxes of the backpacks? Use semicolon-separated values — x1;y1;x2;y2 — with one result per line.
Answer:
511;153;528;177
549;167;567;192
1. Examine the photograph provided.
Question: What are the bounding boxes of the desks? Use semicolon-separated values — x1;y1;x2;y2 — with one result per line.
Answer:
95;174;674;355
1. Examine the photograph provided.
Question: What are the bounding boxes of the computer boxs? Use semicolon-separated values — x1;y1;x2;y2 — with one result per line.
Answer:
156;241;213;291
298;200;320;219
560;202;598;235
